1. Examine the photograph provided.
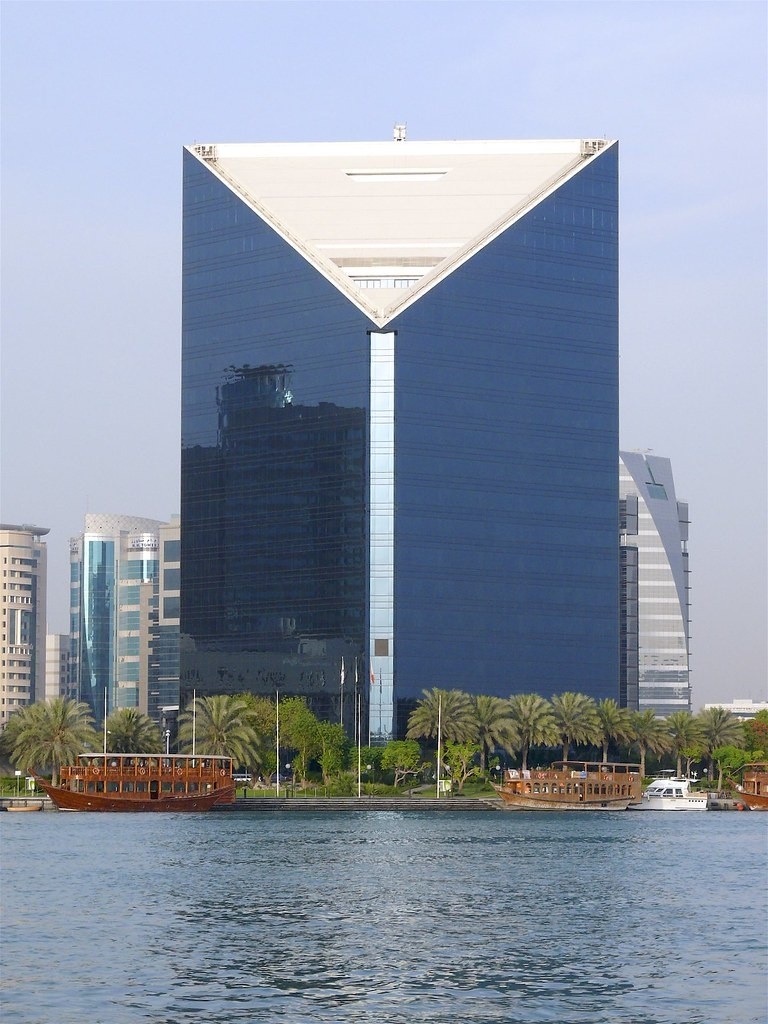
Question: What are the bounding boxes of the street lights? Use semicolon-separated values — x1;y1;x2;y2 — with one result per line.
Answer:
284;764;290;797
165;730;170;768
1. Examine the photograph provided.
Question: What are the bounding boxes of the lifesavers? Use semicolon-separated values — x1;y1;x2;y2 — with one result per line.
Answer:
629;775;634;782
604;774;609;781
177;768;183;776
220;769;226;777
92;767;100;775
140;767;145;775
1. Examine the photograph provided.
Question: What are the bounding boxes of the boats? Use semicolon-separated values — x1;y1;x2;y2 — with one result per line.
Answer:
0;805;40;811
625;779;707;811
489;772;635;810
27;752;236;813
697;789;748;811
725;762;768;811
